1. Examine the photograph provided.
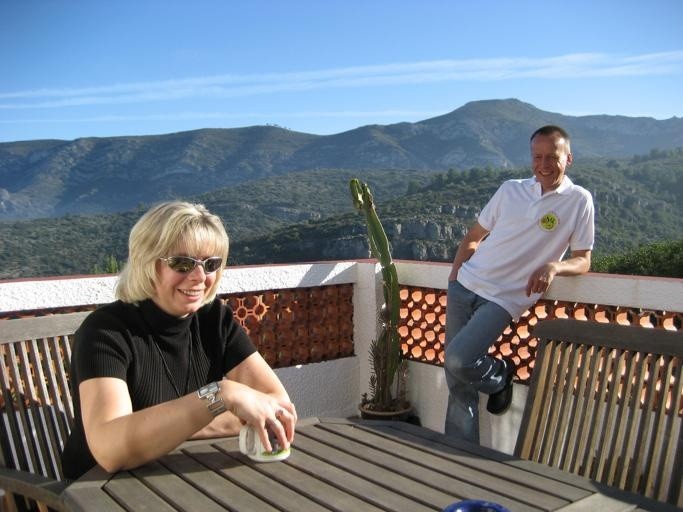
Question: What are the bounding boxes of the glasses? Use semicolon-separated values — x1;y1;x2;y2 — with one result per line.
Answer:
157;256;223;273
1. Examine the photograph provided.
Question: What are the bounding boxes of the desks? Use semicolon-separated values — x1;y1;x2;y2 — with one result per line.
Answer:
51;414;682;511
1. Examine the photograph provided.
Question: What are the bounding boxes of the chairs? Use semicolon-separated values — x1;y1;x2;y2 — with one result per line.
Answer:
510;318;682;510
0;309;100;511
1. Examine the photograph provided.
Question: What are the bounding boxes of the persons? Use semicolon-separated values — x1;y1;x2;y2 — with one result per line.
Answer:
60;201;297;481
443;125;595;447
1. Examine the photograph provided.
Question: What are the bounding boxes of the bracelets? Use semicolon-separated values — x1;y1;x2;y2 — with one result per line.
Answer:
198;383;228;417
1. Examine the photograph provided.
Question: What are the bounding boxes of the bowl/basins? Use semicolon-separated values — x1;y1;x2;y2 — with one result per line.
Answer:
443;500;509;512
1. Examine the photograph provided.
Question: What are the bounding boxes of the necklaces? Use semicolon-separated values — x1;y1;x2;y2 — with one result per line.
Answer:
150;328;194;399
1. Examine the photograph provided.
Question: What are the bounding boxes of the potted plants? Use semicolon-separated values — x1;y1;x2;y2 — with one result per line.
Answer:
349;178;416;420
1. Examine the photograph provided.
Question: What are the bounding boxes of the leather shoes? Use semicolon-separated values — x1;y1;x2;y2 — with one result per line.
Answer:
487;359;516;415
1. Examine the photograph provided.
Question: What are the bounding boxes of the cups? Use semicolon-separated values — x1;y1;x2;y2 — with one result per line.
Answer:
239;420;291;462
